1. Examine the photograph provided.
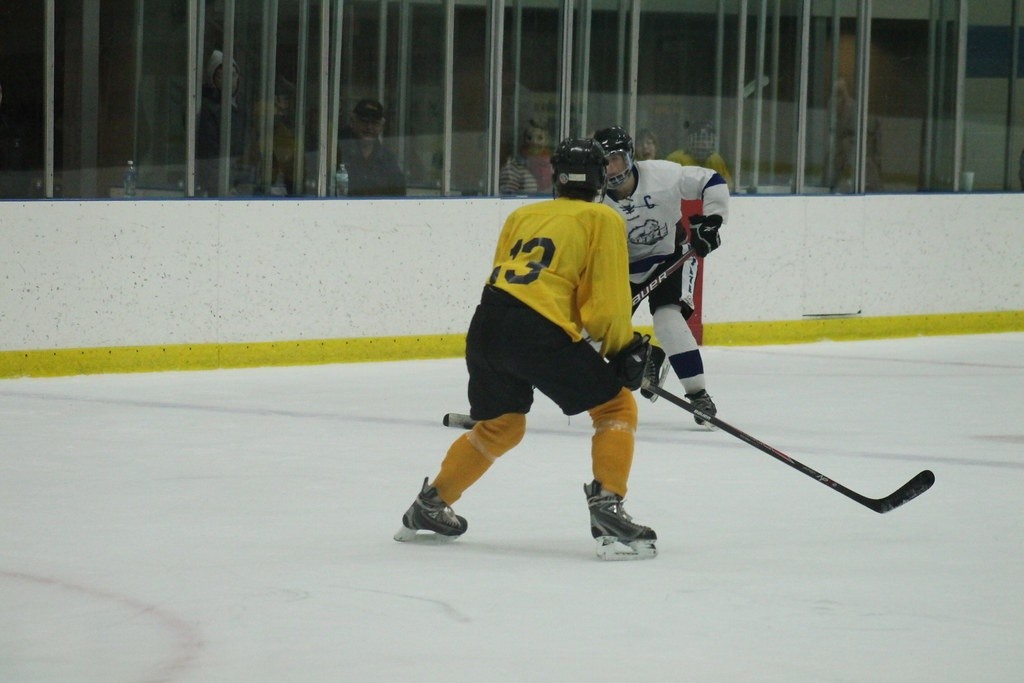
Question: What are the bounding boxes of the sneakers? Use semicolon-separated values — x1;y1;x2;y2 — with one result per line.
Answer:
393;476;468;543
641;343;671;404
583;479;659;561
684;389;720;431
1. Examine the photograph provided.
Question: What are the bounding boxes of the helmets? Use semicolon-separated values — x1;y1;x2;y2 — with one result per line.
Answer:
685;120;718;135
593;125;635;190
549;137;609;204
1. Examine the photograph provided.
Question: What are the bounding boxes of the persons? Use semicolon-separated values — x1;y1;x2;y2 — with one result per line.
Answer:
400;137;670;542
195;48;732;196
594;125;728;431
822;52;897;195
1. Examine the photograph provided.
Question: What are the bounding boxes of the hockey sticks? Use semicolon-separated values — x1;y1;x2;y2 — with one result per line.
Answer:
639;377;936;515
442;241;700;430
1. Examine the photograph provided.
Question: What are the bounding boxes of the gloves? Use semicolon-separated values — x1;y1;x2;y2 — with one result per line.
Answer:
608;331;652;391
687;214;723;257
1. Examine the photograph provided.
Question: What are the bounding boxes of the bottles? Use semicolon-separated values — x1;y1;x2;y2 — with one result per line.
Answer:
123;161;137;198
337;164;348;198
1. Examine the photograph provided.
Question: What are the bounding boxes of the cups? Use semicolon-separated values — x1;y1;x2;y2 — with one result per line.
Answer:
961;172;974;192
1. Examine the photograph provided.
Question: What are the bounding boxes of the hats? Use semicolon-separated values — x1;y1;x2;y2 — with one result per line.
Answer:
354;98;384;120
